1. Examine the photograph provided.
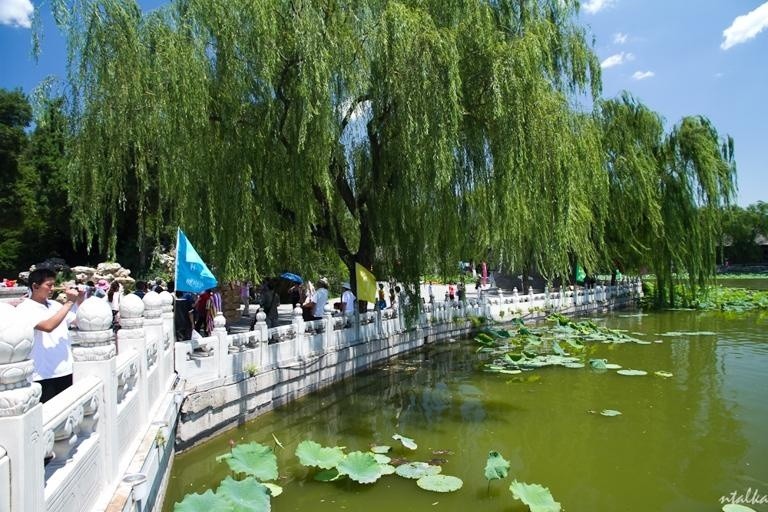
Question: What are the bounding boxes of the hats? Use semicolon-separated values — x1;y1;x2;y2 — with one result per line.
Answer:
342;283;351;289
95;280;108;289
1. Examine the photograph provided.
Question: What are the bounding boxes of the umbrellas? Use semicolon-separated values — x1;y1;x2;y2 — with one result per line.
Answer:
281;272;302;283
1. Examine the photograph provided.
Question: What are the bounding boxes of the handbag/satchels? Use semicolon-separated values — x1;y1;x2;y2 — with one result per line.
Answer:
257;307;270;314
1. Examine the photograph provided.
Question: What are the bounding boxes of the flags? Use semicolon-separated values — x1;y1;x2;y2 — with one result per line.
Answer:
176;228;217;292
356;263;376;304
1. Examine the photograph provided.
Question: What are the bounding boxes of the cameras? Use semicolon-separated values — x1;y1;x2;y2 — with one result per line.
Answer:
70;286;79;291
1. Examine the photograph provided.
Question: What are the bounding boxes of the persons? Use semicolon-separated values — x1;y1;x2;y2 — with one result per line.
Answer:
241;280;251;316
448;279;461;309
388;276;397;288
288;278;329;321
389;289;394;303
63;278;222;341
394;286;401;300
475;273;482;289
16;269;87;403
428;280;435;303
379;283;386;310
0;271;32;306
339;283;356;316
260;282;280;327
